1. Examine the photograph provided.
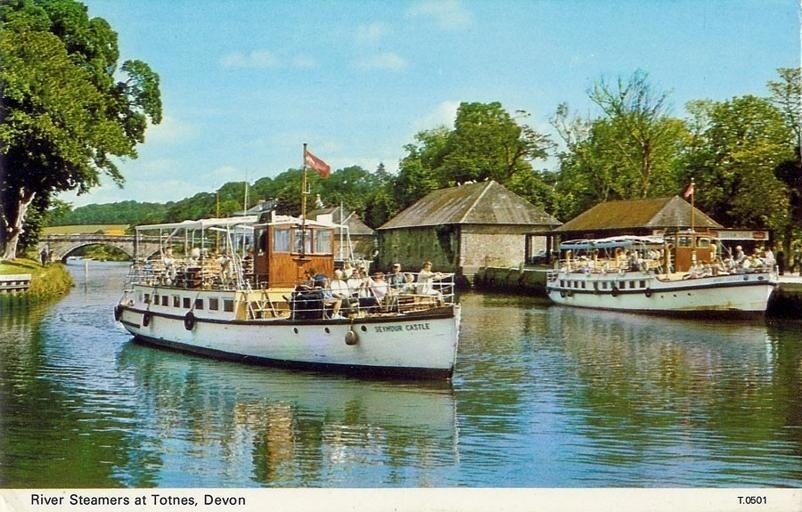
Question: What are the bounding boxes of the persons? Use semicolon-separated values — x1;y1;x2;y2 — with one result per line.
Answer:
40;247;47;267
132;247;442;321
549;244;802;277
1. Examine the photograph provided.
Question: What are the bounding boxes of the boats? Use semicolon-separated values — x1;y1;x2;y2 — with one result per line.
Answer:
115;142;462;377
544;176;780;323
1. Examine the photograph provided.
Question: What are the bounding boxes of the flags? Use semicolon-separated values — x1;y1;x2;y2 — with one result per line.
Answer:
305;150;333;181
686;181;697;198
